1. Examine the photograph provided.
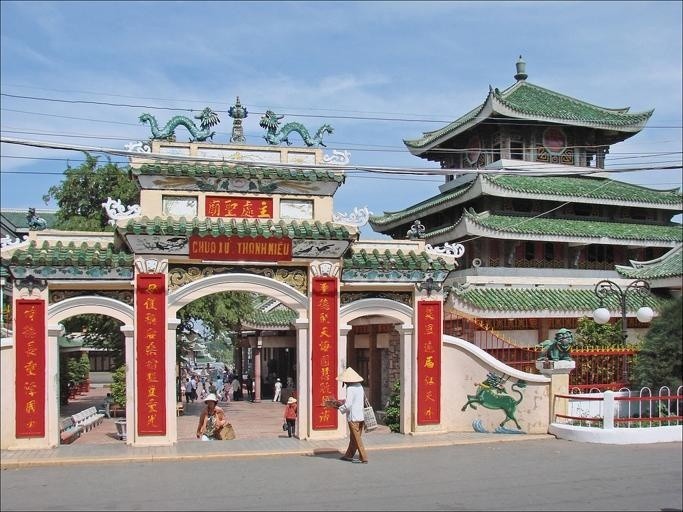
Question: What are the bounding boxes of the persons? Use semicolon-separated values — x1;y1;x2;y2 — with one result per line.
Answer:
196;394;227;441
272;377;282;402
335;366;369;464
282;397;298;439
182;364;255;403
103;392;116;405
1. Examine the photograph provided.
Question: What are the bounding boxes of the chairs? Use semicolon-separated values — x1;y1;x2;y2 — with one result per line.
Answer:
59;406;105;444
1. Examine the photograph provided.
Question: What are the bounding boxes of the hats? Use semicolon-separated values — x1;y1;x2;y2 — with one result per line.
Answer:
203;394;217;401
336;367;364;383
286;397;297;404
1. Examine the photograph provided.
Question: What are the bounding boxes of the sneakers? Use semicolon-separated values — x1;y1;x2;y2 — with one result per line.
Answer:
352;458;368;464
340;456;353;461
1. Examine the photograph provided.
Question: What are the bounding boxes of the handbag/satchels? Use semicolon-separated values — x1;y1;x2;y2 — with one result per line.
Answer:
217;423;236;440
363;406;377;433
283;420;288;431
